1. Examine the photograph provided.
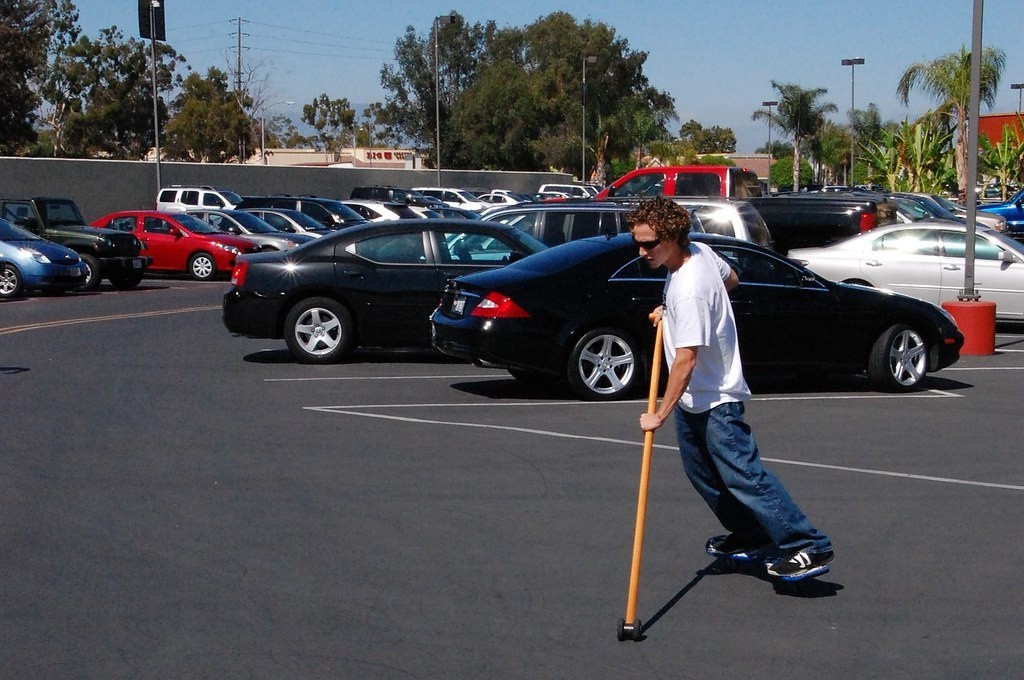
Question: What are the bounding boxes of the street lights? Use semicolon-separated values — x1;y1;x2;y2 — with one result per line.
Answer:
434;14;456;188
762;101;778;196
261;101;296;166
149;0;161;194
1010;81;1024;113
581;55;597;185
840;57;865;189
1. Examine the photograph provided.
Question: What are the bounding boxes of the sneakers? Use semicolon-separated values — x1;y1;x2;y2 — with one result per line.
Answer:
711;533;770;554
767;550;835;576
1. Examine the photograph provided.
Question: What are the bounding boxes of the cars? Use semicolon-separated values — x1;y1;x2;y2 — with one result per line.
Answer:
164;208;317;252
87;209;263;281
0;217;88;298
244;208;333;238
221;219;556;366
426;230;965;402
787;222;1024;323
233;165;1024;266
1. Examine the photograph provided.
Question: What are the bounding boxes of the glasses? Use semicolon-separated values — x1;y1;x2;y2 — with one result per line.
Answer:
632;235;663;250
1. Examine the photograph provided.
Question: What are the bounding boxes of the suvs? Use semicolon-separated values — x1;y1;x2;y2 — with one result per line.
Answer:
0;196;154;292
421;194;702;263
154;183;243;214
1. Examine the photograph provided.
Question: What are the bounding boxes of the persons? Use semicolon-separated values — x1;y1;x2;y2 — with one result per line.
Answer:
622;195;836;575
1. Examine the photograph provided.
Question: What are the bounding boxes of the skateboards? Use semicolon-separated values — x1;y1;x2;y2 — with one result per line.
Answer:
706;532;830;595
